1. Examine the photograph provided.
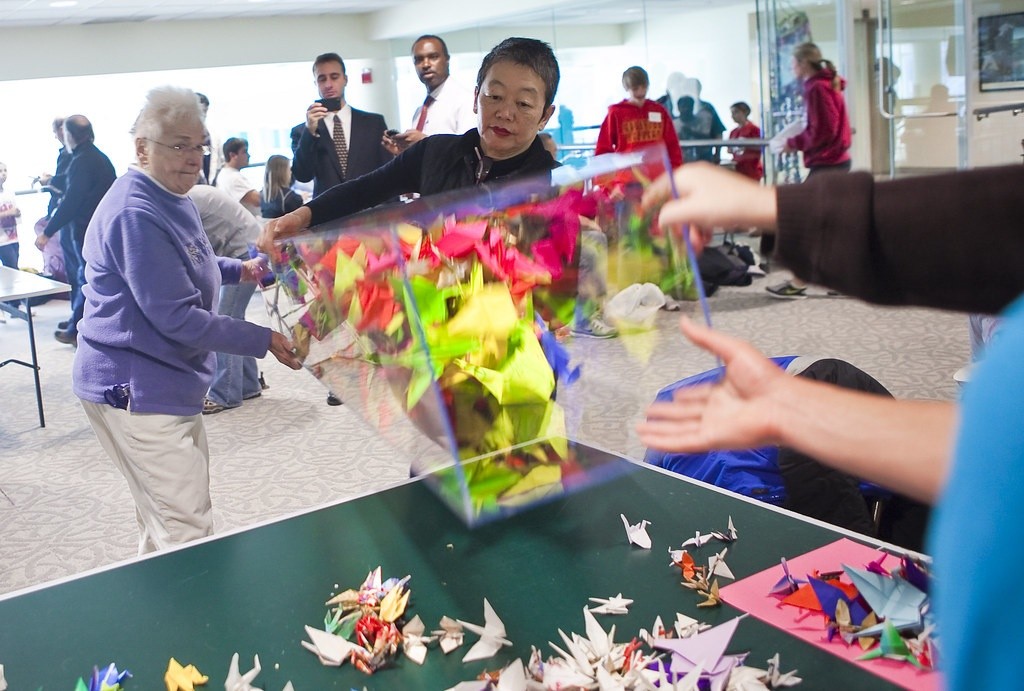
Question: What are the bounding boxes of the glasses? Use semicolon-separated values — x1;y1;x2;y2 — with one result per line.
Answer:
141;137;211;156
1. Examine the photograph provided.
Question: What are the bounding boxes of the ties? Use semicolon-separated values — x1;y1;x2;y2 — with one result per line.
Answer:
333;115;348;181
416;95;434;132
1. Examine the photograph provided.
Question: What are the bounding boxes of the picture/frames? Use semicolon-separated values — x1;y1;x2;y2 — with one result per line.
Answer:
978;12;1024;93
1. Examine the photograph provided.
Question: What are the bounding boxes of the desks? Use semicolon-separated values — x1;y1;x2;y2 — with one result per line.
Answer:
0;436;941;691
0;265;74;430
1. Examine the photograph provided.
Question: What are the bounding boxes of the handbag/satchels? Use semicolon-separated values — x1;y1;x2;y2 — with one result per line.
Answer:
603;283;665;324
643;354;928;554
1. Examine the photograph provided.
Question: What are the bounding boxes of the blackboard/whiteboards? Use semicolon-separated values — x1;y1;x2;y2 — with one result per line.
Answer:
977;9;1023;90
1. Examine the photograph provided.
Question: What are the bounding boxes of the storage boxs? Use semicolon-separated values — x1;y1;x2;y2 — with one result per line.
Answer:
267;143;723;522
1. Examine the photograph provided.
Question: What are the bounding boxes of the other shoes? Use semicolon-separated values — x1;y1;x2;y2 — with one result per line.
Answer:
55;330;77;348
58;321;69;330
327;390;342;406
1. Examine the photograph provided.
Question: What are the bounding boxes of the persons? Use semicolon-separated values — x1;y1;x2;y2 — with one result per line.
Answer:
193;93;212;186
35;114;119;347
764;42;852;299
382;34;479;203
255;38;580;476
0;162;36;323
637;159;1024;691
215;138;260;217
290;53;393;407
538;133;617;339
726;102;763;181
71;85;303;555
188;183;264;415
260;154;302;219
591;66;726;311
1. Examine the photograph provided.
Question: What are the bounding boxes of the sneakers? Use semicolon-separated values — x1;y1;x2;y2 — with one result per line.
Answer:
200;395;225;413
662;295;680;311
827;289;848;296
570;318;618;338
242;390;261;401
766;279;808;299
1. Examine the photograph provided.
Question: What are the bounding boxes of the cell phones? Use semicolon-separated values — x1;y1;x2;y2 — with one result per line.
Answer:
386;129;399;137
314;97;341;112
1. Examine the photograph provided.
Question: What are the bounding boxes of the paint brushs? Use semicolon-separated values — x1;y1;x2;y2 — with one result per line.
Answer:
659;143;723;381
